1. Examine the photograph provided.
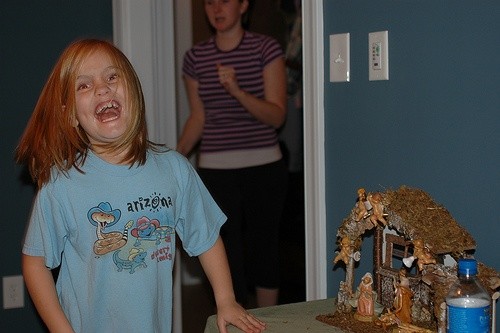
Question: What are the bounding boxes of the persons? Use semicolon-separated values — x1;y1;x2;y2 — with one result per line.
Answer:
393;277;413;323
177;1;287;307
414;239;436;271
356;272;374;316
367;193;387;227
353;187;366;222
18;39;267;333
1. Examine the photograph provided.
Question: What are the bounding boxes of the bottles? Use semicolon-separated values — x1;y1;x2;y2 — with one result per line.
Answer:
444;260;490;332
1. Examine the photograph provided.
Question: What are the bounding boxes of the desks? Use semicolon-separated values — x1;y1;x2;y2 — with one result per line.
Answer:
204;297;400;333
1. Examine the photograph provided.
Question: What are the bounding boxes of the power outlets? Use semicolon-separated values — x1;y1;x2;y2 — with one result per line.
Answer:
2;275;25;309
